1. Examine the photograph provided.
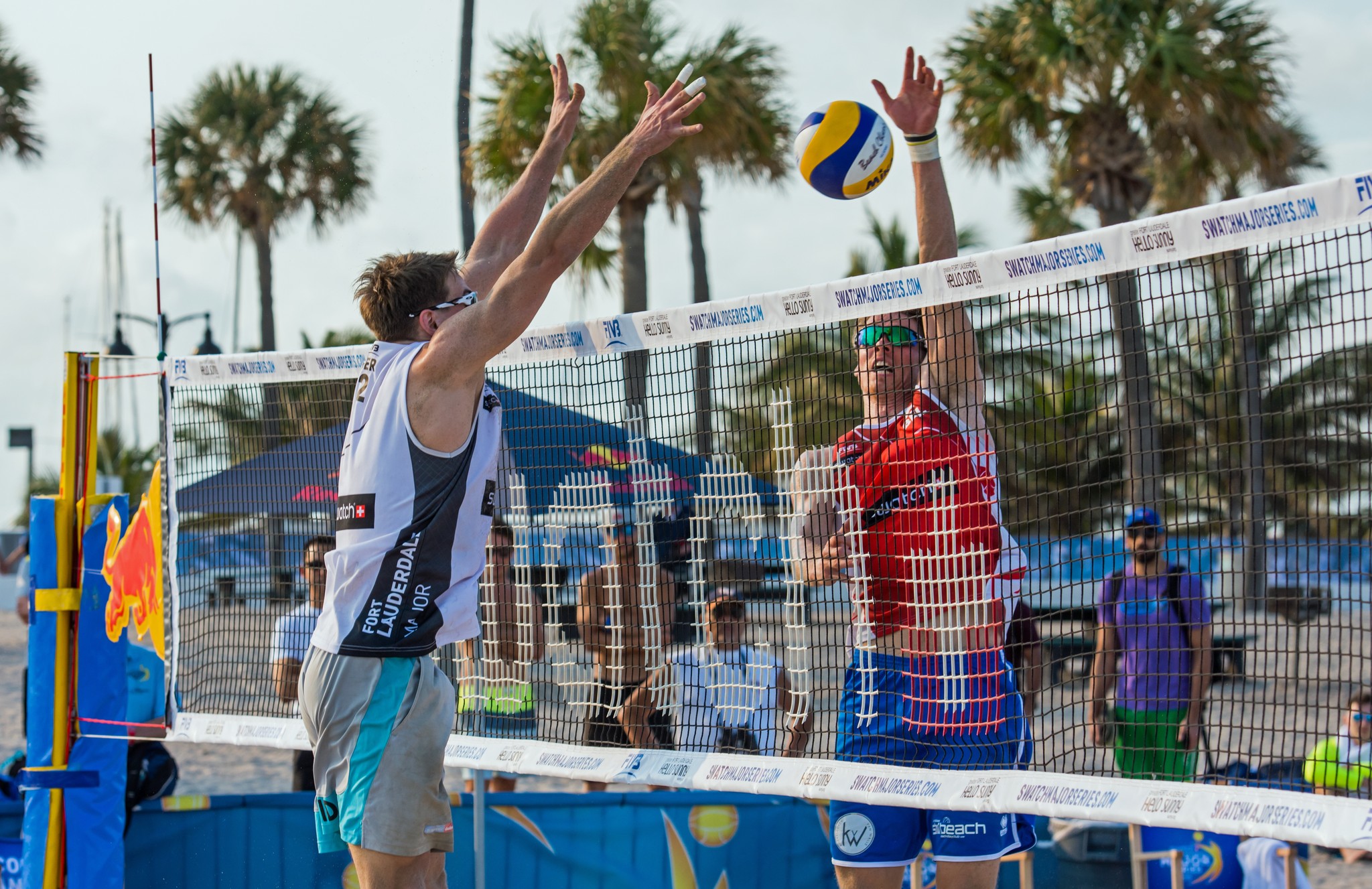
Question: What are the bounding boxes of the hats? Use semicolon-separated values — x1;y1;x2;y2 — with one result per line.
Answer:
608;510;632;524
705;587;741;603
1125;506;1165;536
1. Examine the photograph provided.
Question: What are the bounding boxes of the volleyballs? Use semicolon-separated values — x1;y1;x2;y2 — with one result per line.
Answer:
793;100;894;200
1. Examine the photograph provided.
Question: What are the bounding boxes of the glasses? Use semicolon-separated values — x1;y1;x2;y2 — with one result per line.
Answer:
306;560;326;570
409;289;479;318
486;546;513;557
1127;526;1156;540
606;523;636;539
1352;713;1371;723
854;325;927;352
711;604;742;620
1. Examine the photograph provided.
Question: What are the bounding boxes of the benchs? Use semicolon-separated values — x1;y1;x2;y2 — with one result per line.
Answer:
700;588;803;603
1046;629;1260;694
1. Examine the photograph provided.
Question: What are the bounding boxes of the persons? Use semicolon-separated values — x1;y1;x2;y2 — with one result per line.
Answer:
576;506;681;792
618;586;813;791
268;537;334;792
1086;506;1212;783
0;531;183;799
1302;685;1372;864
454;517;545;792
298;55;708;889
1001;601;1044;726
787;46;1039;889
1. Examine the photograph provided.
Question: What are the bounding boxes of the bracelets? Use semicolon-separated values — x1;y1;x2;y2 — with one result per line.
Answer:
604;615;612;632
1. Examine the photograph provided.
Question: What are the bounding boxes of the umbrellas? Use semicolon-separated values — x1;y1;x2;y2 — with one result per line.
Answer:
176;379;793;521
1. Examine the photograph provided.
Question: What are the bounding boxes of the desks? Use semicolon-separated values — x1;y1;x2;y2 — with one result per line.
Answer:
673;566;812;624
1030;604;1233;679
